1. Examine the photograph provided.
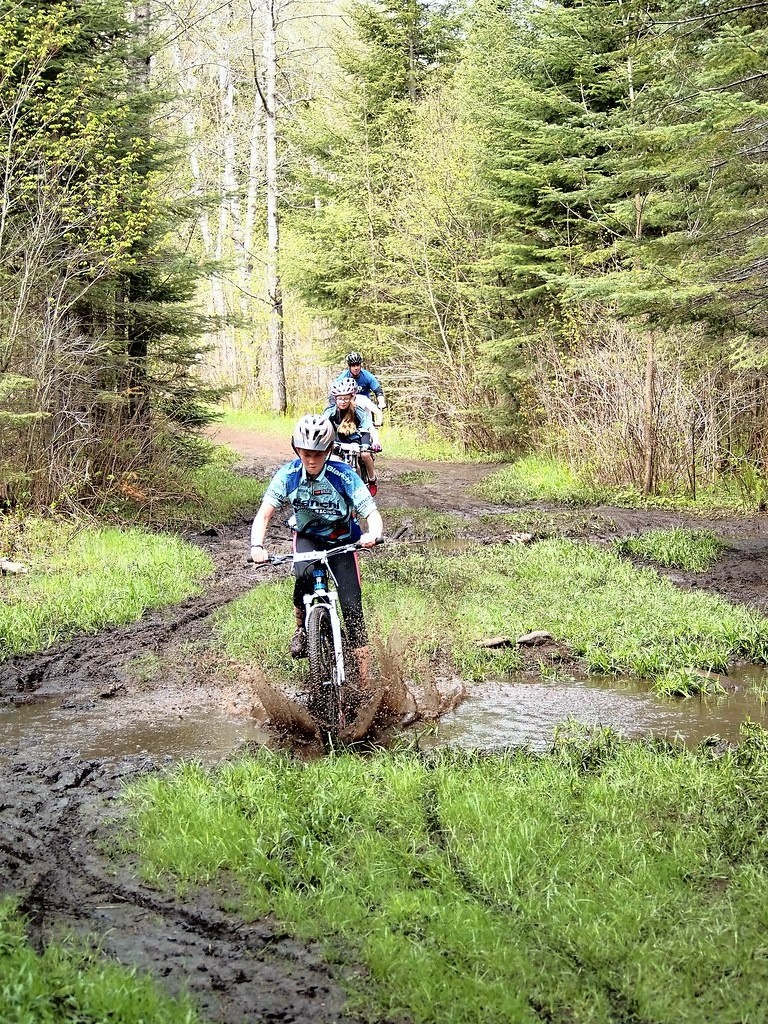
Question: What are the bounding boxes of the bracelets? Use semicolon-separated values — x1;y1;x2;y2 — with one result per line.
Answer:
250;544;263;549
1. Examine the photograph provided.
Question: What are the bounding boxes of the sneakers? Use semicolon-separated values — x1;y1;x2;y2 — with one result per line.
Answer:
368;475;378;497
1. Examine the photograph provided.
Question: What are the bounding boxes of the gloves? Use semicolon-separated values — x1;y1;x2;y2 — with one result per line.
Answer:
372;443;381;452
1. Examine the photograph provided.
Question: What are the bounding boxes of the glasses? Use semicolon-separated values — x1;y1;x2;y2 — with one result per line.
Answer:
335;398;351;402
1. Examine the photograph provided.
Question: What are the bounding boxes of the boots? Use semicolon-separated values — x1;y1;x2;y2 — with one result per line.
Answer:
290;606;307;653
354;645;372;679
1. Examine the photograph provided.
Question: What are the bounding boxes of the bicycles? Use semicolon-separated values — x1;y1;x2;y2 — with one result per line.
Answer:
246;537;385;758
334;403;389;521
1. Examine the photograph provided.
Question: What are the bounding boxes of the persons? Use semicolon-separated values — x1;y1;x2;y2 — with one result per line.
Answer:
250;415;384;693
323;351;388;496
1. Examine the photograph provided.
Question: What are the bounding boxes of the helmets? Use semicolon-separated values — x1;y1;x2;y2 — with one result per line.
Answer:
291;414;335;452
331;381;354;396
339;377;358;394
345;352;363;365
